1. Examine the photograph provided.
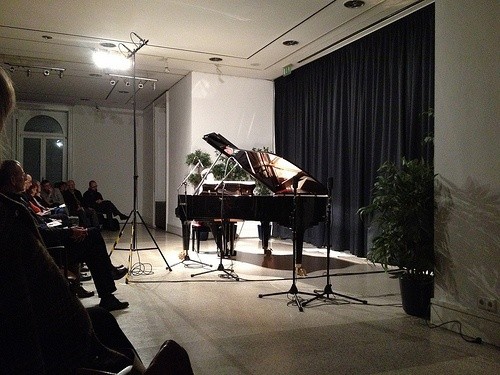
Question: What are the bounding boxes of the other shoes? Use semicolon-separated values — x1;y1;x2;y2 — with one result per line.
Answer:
120;214;130;220
112;267;128;281
99;295;129;310
81;267;89;272
111;263;124;268
72;287;95;298
80;273;91;281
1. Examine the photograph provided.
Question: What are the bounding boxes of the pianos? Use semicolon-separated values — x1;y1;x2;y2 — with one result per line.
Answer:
174;133;330;277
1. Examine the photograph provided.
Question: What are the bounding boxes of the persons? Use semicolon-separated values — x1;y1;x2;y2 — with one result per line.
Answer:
0;65;193;375
203;168;232;259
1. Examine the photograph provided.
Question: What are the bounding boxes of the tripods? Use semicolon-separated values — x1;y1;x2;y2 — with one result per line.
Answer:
190;159;239;283
257;176;326;312
300;178;367;307
165;162;212;272
108;52;171;277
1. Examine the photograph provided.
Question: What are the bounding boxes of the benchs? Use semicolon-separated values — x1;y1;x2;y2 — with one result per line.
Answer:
192;224;236;255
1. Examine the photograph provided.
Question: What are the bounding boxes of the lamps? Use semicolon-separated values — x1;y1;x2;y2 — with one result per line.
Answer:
123;78;130;86
109;76;116;85
138;80;143;88
43;70;49;77
9;66;16;74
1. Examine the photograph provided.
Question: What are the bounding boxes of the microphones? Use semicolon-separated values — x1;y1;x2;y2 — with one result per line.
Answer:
234;159;243;169
198;160;204;168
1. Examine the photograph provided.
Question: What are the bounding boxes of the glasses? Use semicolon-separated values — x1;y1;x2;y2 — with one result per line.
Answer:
15;171;27;178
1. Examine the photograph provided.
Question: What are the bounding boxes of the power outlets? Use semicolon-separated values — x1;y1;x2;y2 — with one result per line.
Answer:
477;296;497;313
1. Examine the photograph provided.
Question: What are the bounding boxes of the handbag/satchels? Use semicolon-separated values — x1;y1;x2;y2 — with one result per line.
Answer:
103;218;120;231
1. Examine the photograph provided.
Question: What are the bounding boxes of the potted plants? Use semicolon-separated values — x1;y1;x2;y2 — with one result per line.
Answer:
186;146;272;241
354;157;434;319
